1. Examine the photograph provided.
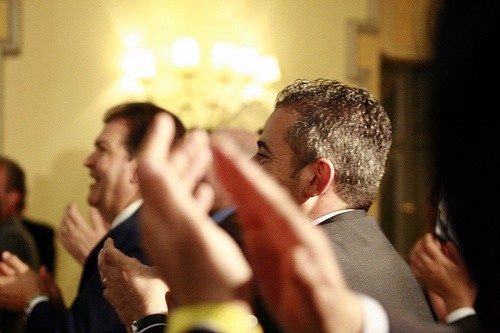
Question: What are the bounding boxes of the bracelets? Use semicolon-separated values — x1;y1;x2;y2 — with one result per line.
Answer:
166;299;264;333
130;312;167;333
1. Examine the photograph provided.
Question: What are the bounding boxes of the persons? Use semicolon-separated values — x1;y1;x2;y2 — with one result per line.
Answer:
0;0;500;333
